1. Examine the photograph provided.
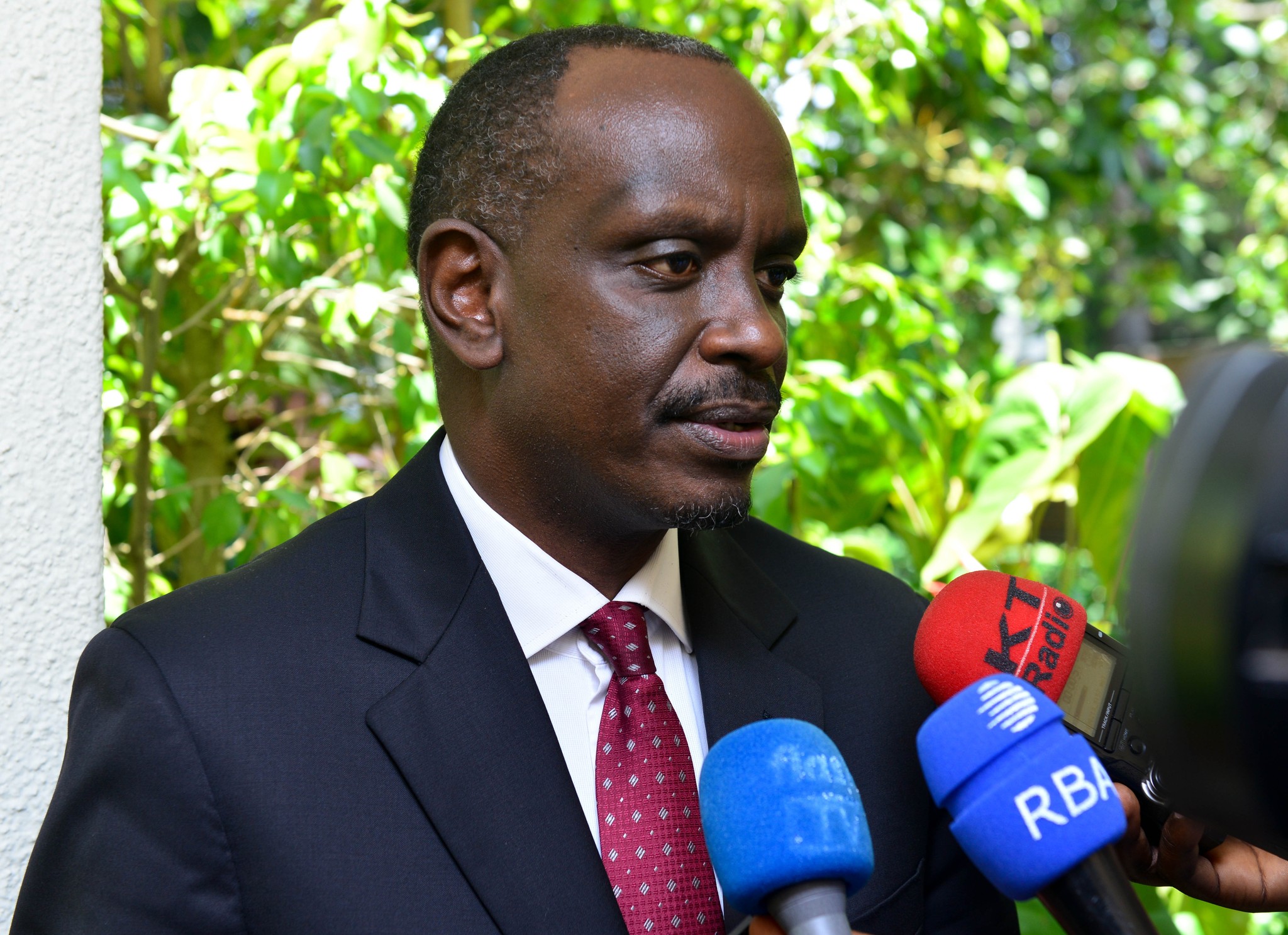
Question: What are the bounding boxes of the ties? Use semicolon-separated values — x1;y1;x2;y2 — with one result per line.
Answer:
580;601;728;935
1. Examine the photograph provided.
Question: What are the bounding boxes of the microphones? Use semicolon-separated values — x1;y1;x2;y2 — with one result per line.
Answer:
907;566;1252;855
916;671;1161;934
695;715;873;935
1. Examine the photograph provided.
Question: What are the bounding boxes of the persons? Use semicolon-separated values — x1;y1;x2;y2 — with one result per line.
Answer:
1084;728;1288;920
6;24;1036;935
